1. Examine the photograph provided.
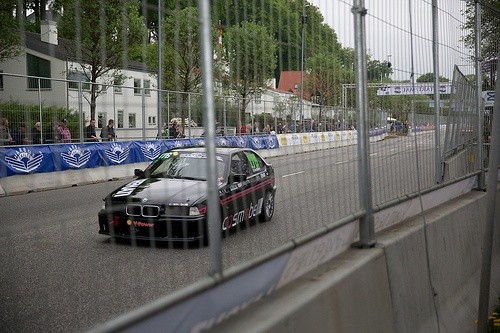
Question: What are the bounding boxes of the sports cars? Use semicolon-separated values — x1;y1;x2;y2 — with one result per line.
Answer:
98;146;278;247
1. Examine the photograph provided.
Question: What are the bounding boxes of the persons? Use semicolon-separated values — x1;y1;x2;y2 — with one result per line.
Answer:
156;121;186;139
240;118;409;135
0;118;118;146
